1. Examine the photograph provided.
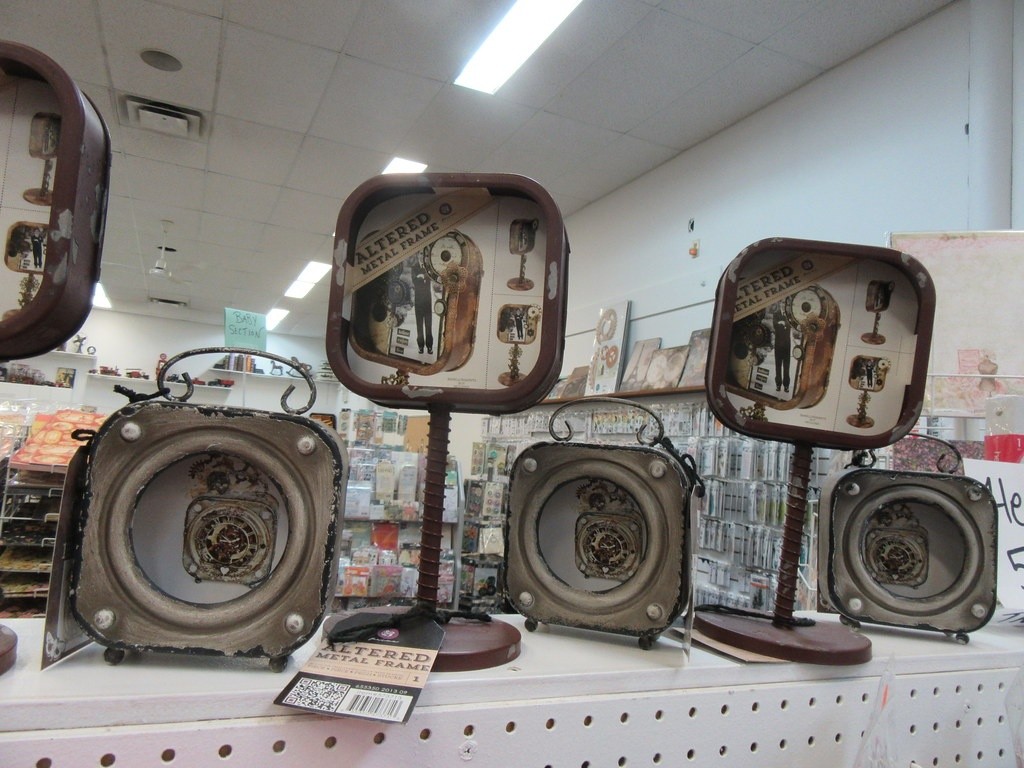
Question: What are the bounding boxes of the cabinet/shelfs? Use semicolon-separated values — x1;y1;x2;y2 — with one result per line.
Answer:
0;437;69;597
334;444;462;613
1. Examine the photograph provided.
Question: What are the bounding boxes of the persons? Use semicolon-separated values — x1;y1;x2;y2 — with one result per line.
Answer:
866;359;874;388
514;306;524;341
772;297;795;394
411;248;435;355
30;227;44;269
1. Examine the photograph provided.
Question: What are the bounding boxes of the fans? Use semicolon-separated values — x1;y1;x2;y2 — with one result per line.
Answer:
108;219;187;285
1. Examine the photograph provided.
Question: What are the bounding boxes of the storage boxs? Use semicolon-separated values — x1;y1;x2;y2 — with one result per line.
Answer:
340;449;454;605
